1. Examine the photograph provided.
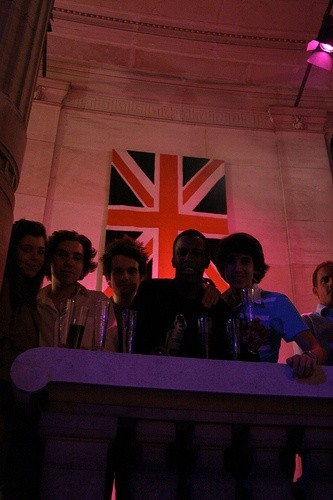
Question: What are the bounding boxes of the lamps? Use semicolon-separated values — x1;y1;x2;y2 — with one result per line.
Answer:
307;14;333;71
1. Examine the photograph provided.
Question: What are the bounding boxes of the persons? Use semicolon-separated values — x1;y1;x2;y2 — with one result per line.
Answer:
0;219;49;360
102;238;148;353
34;230;119;353
294;260;333;367
133;228;232;359
201;232;327;378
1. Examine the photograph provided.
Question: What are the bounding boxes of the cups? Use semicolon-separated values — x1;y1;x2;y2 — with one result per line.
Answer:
37;294;139;355
240;289;256;324
224;318;241;361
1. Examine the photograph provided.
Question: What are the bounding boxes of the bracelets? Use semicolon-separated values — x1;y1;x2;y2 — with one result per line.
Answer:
302;350;320;363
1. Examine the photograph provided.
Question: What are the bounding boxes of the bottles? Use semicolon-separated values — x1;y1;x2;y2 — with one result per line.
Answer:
195;306;212;358
